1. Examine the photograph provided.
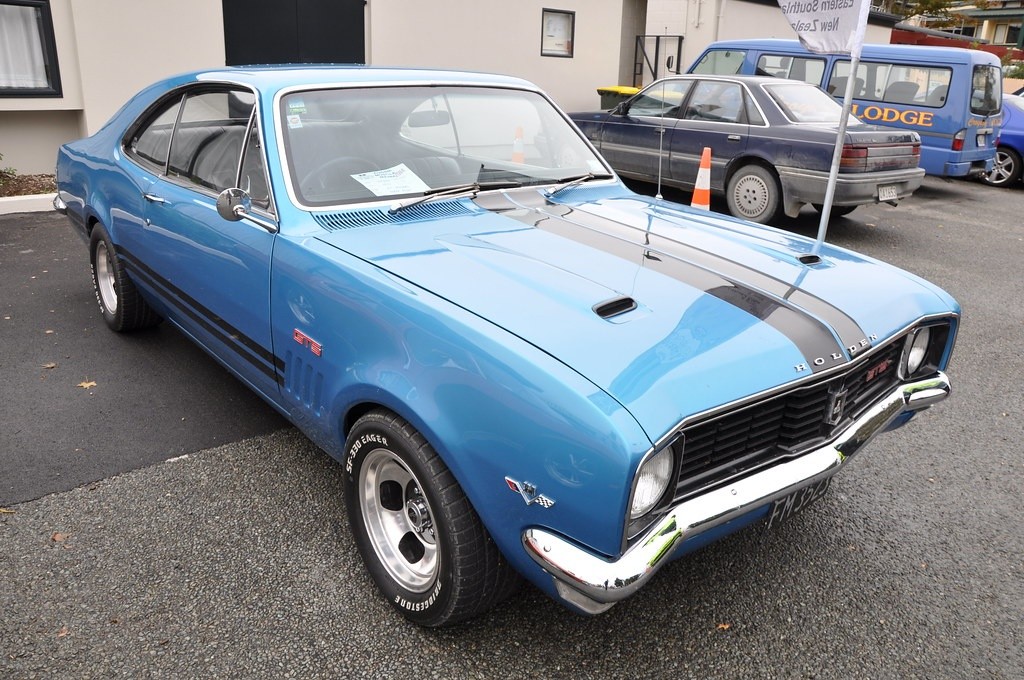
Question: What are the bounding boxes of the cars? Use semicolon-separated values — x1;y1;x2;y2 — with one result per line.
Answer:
565;72;925;225
912;85;1024;188
52;62;963;627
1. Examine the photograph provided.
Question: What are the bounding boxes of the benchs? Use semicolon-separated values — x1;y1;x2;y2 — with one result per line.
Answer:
135;120;364;200
828;77;864;96
928;85;948;105
884;81;920;101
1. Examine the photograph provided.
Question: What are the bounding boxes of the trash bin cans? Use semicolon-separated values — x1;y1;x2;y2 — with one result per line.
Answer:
598;86;640;109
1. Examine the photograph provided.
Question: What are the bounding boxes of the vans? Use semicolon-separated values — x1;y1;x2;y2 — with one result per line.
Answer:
665;39;1003;178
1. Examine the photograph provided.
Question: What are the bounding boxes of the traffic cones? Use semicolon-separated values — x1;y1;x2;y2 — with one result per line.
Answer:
509;124;527;163
692;145;711;212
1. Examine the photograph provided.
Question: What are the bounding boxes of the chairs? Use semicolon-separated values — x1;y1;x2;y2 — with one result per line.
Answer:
776;57;790;78
756;57;766;75
